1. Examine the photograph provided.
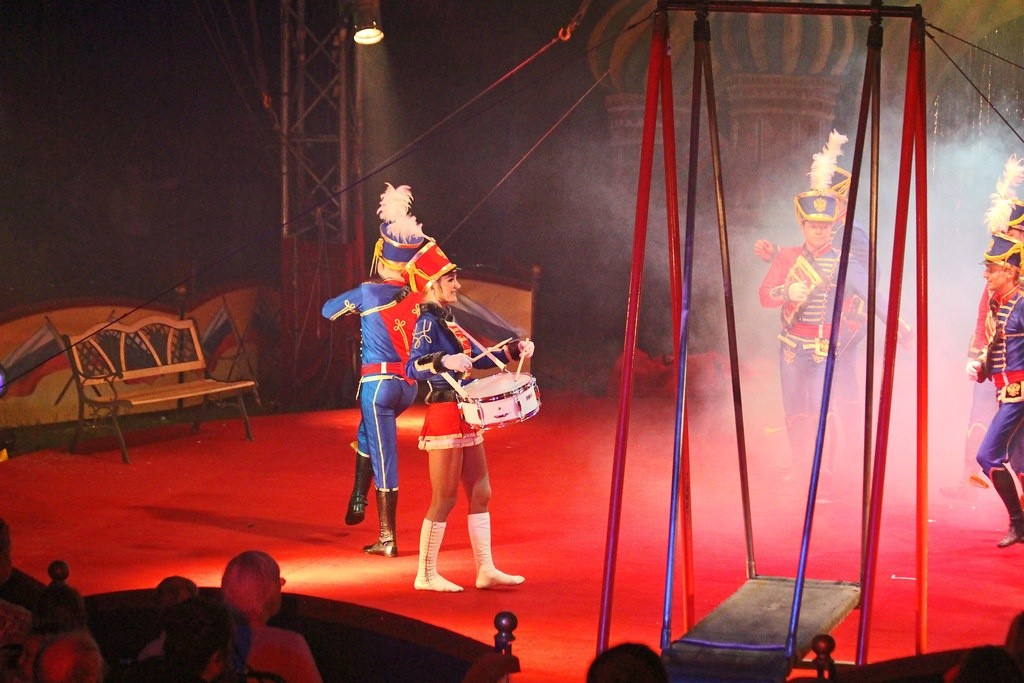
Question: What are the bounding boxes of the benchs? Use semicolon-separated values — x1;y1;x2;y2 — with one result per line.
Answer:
61;316;260;464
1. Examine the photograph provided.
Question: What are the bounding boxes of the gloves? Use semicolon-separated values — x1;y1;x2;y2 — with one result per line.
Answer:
899;319;908;337
517;340;534;358
442;353;475;373
967;360;983;382
789;282;810;304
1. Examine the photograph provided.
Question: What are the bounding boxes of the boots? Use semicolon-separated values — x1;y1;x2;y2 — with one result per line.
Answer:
414;518;464;592
344;448;374;526
361;487;399;558
468;512;524;588
983;460;1023;547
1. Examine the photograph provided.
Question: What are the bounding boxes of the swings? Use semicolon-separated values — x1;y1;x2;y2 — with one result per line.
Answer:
657;12;894;679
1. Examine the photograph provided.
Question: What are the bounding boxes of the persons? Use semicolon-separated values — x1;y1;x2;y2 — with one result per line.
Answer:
939;603;1024;683
322;220;470;557
752;162;922;509
967;199;1024;489
963;232;1024;550
0;517;326;683
405;239;535;592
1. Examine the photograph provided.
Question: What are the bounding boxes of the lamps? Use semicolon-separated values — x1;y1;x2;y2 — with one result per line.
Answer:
350;0;385;45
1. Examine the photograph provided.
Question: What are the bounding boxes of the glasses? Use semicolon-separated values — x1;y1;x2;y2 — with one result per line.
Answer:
275;578;286;590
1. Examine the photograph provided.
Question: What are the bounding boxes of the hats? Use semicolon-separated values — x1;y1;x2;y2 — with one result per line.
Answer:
793;152;838;224
978;200;1024;275
813;128;851;202
990;153;1024;231
387;216;461;294
375;182;424;261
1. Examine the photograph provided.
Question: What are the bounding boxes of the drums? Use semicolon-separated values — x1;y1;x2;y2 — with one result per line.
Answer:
457;371;541;429
